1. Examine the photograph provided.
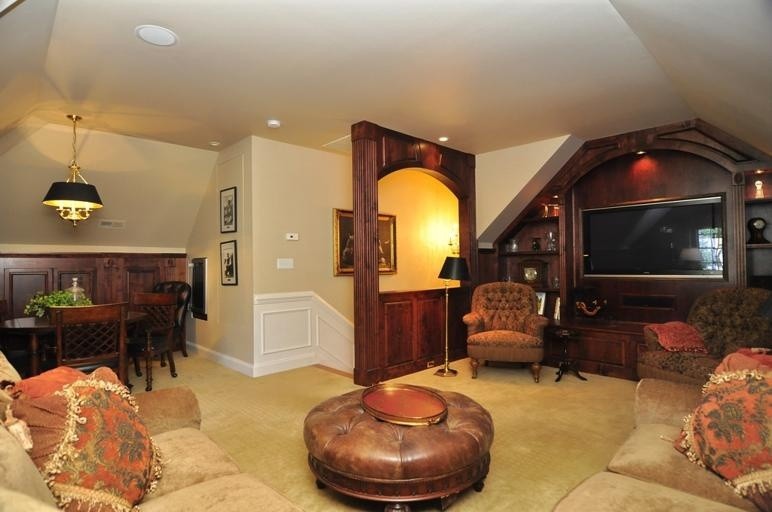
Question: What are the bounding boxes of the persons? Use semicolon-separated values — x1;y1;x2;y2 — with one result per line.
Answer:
224;253;232;278
224;200;232;224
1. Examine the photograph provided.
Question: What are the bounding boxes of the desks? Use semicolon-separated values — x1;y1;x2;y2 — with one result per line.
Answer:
1;311;150;389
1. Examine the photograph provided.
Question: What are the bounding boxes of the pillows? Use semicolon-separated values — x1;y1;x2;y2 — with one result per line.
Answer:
0;366;161;511
659;346;771;512
647;321;708;353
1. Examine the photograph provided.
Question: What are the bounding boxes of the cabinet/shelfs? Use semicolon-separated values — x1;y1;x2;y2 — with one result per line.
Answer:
542;330;645;381
732;169;772;289
499;193;561;328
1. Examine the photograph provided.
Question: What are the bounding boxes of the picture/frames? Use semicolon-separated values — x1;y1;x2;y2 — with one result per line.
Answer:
332;207;398;276
219;186;237;233
220;240;238;286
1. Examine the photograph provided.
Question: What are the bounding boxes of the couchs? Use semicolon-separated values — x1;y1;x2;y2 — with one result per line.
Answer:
303;383;496;512
548;379;770;512
462;280;551;383
635;287;771;385
0;351;304;512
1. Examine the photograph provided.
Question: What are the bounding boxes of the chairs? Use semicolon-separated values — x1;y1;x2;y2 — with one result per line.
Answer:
140;280;191;358
48;301;131;390
129;291;179;392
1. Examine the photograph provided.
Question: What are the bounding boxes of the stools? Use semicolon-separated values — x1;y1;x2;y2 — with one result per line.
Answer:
551;327;588;382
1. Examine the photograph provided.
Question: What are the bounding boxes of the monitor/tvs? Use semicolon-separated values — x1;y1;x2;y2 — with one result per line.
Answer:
578;195;725;279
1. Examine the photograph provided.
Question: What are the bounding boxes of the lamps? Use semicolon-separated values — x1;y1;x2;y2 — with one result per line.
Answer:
42;115;105;228
434;256;472;377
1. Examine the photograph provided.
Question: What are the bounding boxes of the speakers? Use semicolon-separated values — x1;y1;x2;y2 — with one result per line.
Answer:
732;171;745;185
